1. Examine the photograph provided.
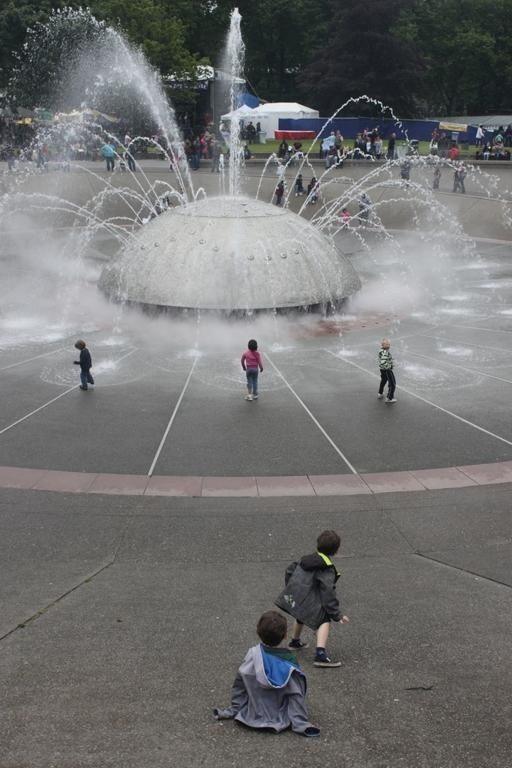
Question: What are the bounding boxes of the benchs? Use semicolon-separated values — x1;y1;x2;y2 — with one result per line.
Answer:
475;151;512;160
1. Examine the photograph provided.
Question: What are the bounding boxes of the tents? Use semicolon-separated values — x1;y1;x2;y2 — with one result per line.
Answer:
254;102;320;140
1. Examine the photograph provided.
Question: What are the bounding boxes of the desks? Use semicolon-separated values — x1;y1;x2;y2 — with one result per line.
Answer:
274;129;317;139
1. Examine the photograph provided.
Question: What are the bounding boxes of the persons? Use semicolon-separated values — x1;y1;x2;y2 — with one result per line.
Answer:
241;340;264;401
212;609;320;738
273;529;351;668
376;338;398;403
0;120;511;231
71;340;94;391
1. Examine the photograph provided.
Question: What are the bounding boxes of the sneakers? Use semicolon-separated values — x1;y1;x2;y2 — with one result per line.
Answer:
377;392;385;399
244;394;253;401
253;394;259;399
289;639;309;651
313;655;342;668
87;379;94;384
80;384;88;390
384;397;397;403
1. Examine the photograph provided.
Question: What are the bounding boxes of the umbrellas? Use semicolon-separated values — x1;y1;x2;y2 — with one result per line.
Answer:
221;105;269;120
